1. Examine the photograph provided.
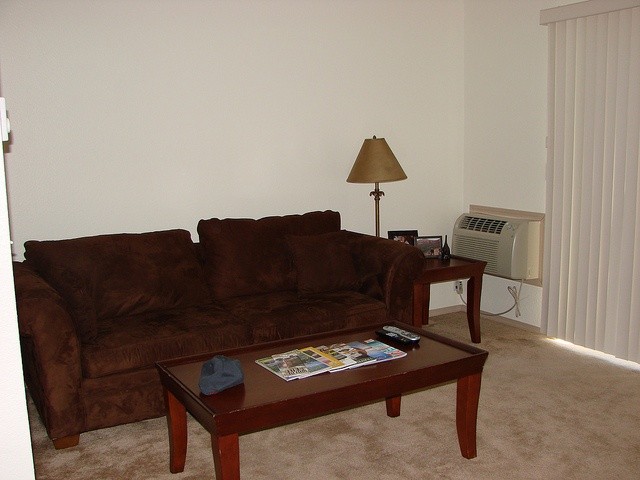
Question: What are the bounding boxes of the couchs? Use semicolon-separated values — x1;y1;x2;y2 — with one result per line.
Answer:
13;211;425;449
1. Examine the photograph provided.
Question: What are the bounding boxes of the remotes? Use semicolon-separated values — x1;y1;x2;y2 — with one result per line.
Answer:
382;325;421;343
375;329;411;345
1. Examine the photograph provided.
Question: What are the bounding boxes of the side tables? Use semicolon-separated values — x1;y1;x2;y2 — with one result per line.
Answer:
422;254;487;343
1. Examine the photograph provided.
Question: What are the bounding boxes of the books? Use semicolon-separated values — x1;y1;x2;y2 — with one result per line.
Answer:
255;348;332;382
361;338;408;365
314;345;357;369
297;346;345;379
328;342;376;373
346;340;393;369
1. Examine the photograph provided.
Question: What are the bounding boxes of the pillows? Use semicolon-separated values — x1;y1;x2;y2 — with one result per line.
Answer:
285;231;361;297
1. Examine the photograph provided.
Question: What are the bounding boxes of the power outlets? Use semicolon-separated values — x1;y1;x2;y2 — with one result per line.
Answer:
455;281;463;292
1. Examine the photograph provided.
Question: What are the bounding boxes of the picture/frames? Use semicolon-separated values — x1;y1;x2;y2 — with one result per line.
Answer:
388;230;418;246
414;235;442;258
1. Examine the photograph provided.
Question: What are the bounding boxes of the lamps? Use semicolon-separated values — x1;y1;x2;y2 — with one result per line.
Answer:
345;136;407;238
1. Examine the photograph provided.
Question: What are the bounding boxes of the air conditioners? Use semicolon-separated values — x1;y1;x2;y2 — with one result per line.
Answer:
451;206;540;283
